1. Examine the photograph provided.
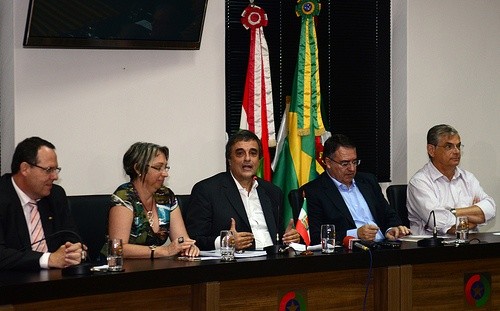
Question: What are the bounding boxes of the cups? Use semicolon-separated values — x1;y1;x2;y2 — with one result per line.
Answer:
107;238;123;271
220;230;235;260
455;217;470;243
321;225;335;253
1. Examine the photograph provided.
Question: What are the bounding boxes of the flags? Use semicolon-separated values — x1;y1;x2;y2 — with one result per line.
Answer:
239;6;277;181
270;0;327;230
295;198;311;246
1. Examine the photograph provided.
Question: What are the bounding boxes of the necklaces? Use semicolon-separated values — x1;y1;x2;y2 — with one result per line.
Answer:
141;196;154;228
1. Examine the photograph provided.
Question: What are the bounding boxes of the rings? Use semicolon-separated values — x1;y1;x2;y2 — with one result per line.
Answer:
297;235;298;237
178;237;184;243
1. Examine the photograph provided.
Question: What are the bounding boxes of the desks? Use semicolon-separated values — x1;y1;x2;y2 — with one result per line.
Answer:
0;231;500;311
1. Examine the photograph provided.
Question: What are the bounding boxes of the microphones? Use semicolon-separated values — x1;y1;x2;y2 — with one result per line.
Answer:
251;175;287;254
341;235;371;251
417;210;444;247
0;229;93;277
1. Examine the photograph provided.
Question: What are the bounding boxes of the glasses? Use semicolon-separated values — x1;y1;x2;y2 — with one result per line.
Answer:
326;156;361;167
433;143;465;150
27;162;61;175
147;164;170;172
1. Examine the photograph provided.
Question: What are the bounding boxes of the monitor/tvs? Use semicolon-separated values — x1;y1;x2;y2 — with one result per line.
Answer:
22;0;209;51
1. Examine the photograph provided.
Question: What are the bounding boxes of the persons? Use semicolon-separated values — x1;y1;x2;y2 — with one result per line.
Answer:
298;133;413;241
405;124;497;236
186;129;300;250
0;135;88;270
100;141;200;264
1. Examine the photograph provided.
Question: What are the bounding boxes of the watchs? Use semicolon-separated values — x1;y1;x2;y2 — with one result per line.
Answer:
450;208;456;215
150;245;156;259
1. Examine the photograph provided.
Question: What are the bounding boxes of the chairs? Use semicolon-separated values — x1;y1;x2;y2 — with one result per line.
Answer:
386;185;411;229
65;194;113;263
174;194;191;226
288;189;304;227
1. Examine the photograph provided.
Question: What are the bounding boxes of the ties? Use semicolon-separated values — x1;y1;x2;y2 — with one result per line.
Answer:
27;199;48;253
